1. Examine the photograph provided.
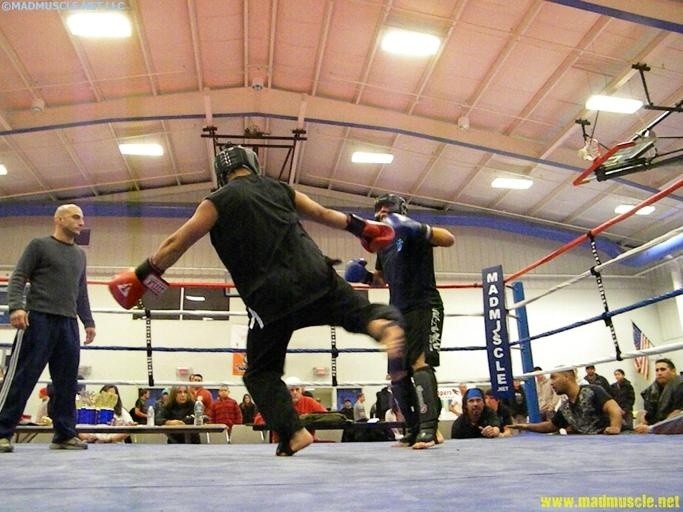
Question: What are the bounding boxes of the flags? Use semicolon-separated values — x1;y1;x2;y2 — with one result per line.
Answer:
632;322;650;382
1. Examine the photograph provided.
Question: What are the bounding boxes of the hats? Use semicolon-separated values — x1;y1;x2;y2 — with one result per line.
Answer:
162;389;170;396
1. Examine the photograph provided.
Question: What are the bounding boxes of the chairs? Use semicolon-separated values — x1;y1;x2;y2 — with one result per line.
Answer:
130;432;168;445
230;425;264;444
314;430;344;442
199;432;229;444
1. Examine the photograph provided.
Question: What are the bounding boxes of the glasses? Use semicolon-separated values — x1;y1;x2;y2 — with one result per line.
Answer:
655;366;667;372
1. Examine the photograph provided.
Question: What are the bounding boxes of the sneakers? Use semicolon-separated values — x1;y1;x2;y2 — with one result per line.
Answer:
50;436;88;450
0;438;14;452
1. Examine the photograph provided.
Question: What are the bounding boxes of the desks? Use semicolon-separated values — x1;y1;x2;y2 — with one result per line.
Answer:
14;424;230;444
252;421;406;443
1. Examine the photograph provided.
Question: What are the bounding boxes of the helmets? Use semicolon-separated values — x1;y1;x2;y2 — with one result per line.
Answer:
374;194;409;221
214;145;261;186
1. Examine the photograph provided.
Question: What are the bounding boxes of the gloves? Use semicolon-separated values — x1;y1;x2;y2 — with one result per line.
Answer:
345;213;395;254
107;256;169;310
383;212;433;242
344;257;374;285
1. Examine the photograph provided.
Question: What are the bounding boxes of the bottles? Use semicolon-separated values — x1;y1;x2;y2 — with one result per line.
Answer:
146;405;155;427
193;397;204;425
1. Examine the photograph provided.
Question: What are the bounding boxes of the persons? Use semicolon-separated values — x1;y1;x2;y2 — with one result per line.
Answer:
483;390;519;436
154;392;171;413
584;365;609;391
345;193;455;449
135;389;153;424
340;398;355;443
109;146;406;457
353;393;369;423
239;394;256;423
155;385;208;444
634;359;683;434
36;388;50;422
452;388;502;440
206;385;243;433
449;383;467;417
0;204;95;453
533;366;559;435
188;374;212;410
254;377;328;443
506;365;622;435
608;369;635;431
512;378;528;424
385;396;408;441
78;385;133;444
